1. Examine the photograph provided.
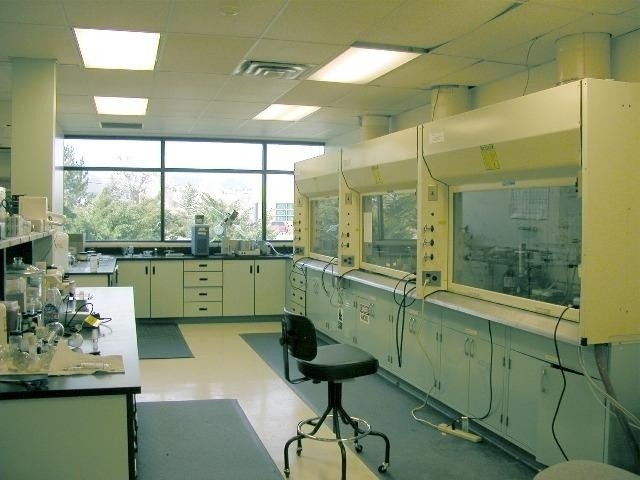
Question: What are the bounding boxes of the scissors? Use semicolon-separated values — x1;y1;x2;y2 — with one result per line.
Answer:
1;196;15;215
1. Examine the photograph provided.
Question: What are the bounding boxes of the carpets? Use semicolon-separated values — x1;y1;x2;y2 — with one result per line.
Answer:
137;320;194;358
237;327;540;479
137;400;288;479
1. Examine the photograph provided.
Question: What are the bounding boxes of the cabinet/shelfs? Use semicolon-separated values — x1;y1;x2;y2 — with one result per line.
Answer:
184;260;224;317
304;267;330;346
392;292;439;399
287;260;307;315
438;308;514;437
116;259;184;318
222;259;286;316
504;324;610;472
350;281;392;371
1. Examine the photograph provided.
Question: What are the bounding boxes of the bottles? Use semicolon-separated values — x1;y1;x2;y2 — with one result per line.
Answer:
0;250;105;373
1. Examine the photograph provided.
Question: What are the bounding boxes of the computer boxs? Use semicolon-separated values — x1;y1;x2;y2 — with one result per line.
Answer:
191;224;209;256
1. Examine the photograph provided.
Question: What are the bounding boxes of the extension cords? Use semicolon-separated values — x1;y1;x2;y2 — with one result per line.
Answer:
437;422;482;443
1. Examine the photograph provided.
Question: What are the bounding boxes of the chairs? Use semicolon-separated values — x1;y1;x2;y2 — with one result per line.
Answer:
278;311;390;480
